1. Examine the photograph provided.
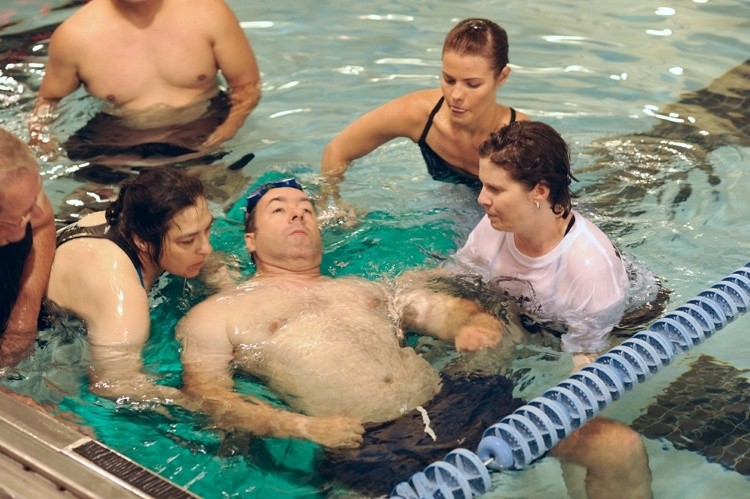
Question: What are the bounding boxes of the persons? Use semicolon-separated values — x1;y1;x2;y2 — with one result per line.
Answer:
431;121;670;376
0;122;56;383
34;166;238;423
24;0;261;148
316;19;532;231
175;179;653;499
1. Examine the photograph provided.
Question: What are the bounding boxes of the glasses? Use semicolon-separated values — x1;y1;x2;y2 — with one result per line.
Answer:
246;177;296;204
0;181;44;227
